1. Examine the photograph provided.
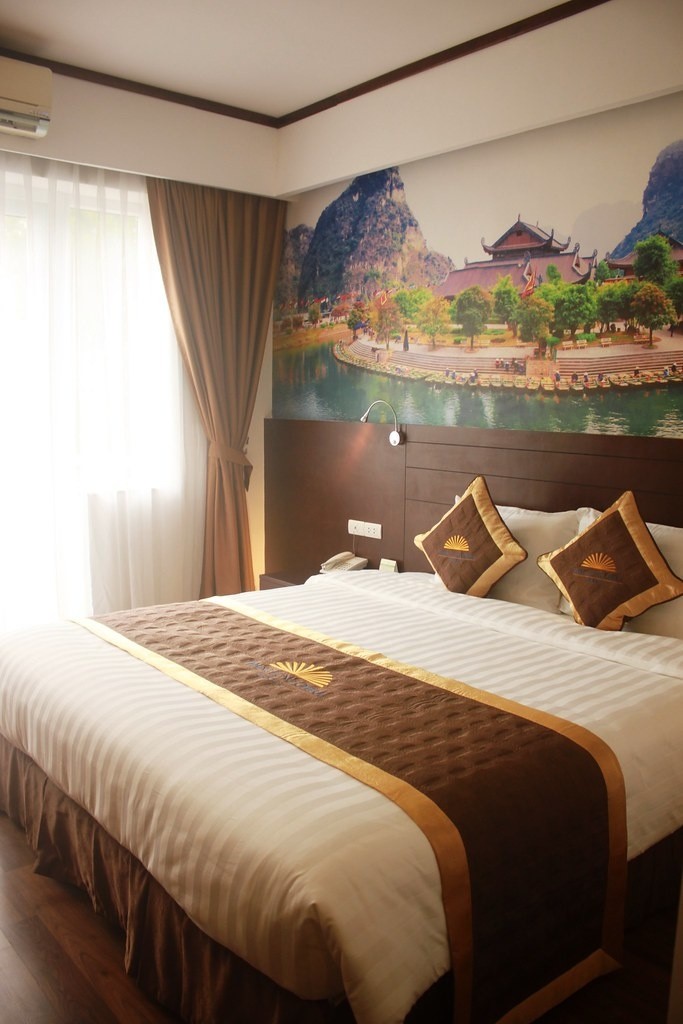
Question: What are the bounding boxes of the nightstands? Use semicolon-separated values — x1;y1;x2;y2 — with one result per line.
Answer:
259;570;318;590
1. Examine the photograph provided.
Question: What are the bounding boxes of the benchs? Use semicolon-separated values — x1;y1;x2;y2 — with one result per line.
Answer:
576;339;588;349
479;339;491;349
633;334;654;344
562;340;574;350
599;337;612;347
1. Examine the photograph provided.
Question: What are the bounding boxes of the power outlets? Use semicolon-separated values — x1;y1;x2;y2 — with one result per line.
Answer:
347;519;383;540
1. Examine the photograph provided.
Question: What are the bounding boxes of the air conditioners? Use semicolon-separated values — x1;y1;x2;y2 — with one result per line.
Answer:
0;55;54;141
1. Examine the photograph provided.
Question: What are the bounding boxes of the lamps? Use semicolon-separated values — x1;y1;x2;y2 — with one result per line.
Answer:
360;400;405;446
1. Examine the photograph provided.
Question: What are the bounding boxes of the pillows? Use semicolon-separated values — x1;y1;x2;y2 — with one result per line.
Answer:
537;489;683;630
558;507;683;638
454;494;576;614
413;476;528;597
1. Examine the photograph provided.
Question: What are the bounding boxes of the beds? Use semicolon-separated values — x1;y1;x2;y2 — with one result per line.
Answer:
0;417;683;1024
